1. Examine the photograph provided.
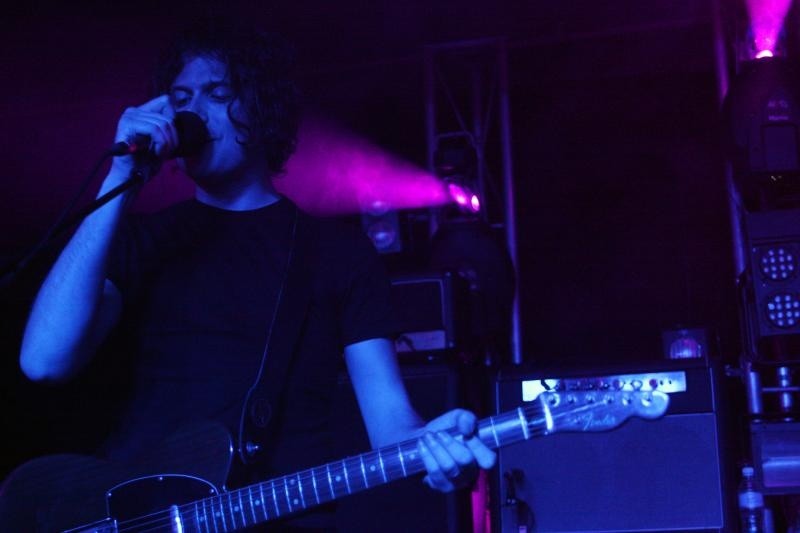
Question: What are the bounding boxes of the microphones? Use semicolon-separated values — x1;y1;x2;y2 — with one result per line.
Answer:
112;111;209;156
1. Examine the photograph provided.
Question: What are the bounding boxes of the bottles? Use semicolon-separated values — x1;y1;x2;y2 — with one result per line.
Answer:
738;467;766;533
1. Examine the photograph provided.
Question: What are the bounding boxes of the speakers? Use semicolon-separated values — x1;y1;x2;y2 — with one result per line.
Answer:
492;369;725;533
246;365;464;533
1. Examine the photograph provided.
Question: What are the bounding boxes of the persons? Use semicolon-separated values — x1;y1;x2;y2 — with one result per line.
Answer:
0;33;498;533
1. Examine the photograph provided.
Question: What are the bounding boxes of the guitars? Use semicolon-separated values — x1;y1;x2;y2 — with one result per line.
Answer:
0;376;672;533
